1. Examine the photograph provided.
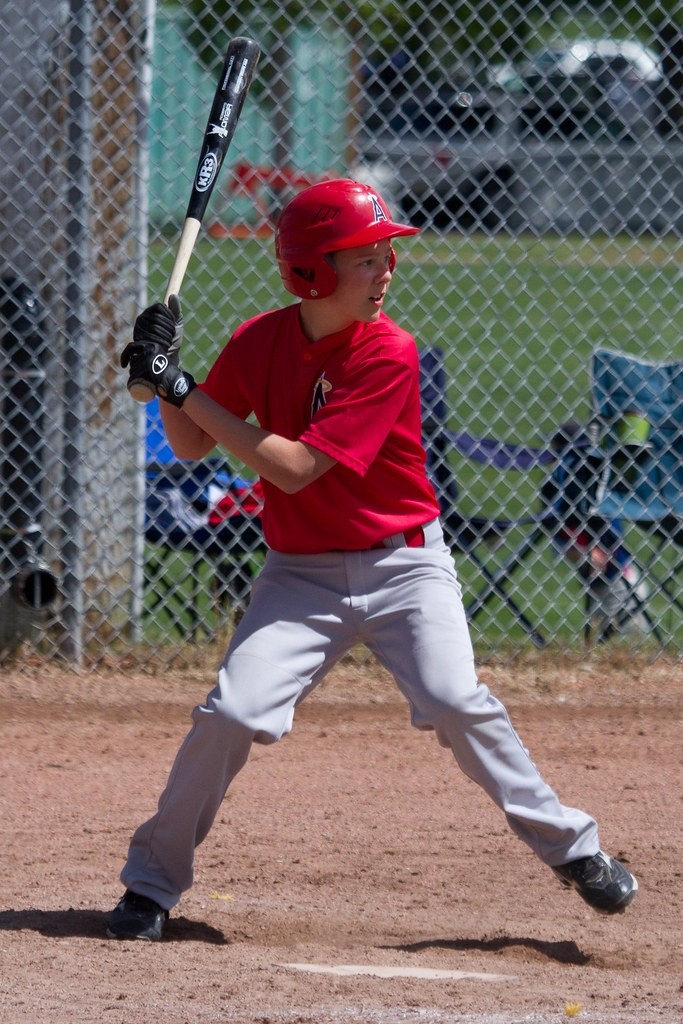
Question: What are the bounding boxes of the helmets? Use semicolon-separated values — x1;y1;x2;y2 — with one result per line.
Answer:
274;178;420;300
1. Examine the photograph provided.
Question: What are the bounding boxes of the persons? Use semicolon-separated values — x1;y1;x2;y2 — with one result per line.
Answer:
540;423;652;649
108;179;638;941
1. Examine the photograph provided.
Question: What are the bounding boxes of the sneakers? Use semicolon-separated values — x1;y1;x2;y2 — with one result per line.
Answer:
552;848;638;915
106;888;170;942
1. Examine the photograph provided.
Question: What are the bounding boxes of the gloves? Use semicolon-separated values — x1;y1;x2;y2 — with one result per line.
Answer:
121;294;201;410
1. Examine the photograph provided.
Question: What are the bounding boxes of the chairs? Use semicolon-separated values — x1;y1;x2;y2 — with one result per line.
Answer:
419;345;593;650
142;394;267;644
585;348;683;642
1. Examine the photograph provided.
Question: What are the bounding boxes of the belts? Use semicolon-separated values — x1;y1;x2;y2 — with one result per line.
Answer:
374;525;426;548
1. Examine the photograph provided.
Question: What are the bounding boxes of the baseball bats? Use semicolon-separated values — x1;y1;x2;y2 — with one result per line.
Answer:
126;36;262;404
